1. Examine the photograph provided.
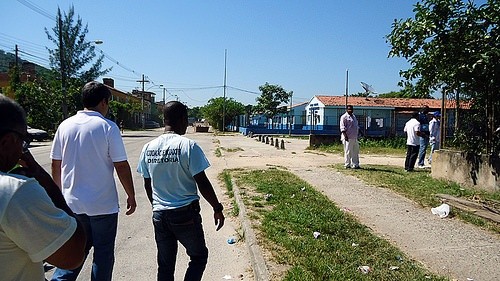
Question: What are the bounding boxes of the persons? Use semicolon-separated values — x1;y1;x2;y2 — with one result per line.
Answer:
50;80;137;281
417;115;430;169
428;112;441;163
137;101;225;281
0;92;91;281
403;112;429;172
339;104;364;169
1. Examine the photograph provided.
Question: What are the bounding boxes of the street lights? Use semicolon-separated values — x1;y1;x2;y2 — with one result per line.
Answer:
310;103;319;134
141;84;164;130
163;94;177;127
60;40;103;120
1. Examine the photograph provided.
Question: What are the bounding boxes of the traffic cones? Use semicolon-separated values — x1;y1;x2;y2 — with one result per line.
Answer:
261;136;265;143
258;135;261;141
265;136;269;144
280;139;285;149
269;137;274;146
274;139;279;147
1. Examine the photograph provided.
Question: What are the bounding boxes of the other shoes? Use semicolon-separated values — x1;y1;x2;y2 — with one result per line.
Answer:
354;166;364;169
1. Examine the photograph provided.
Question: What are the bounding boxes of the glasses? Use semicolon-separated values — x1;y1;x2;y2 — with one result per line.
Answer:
7;127;33;152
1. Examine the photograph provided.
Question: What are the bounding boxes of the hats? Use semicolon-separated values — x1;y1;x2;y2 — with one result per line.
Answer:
434;111;442;117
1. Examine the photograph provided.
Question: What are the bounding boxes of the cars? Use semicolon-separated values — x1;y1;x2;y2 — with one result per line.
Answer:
26;125;49;142
145;121;158;129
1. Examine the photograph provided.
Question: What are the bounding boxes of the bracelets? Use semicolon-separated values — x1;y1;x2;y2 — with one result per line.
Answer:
213;202;223;212
